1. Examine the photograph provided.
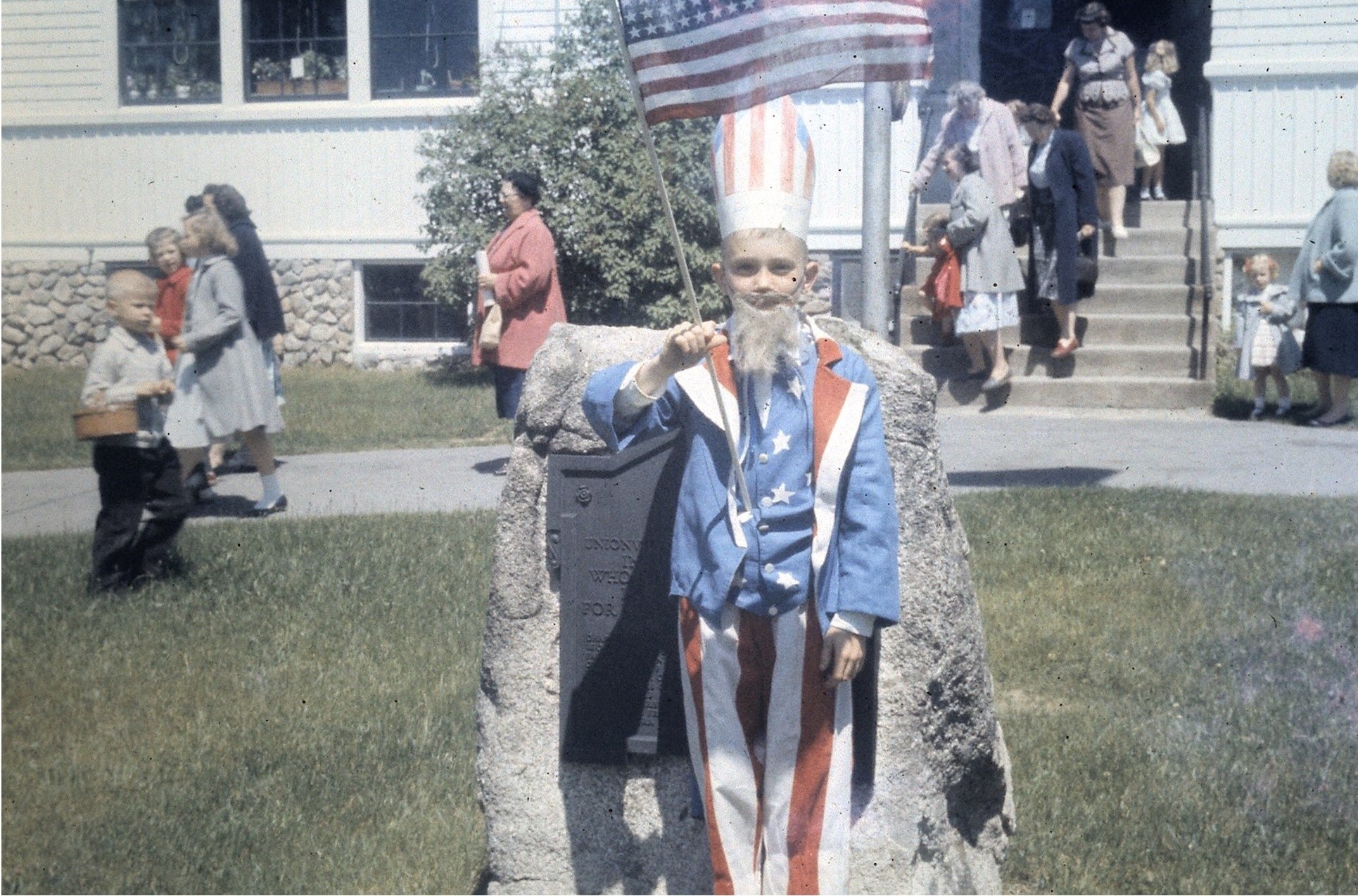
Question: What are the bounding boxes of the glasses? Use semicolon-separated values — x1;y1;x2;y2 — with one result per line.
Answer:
497;190;528;202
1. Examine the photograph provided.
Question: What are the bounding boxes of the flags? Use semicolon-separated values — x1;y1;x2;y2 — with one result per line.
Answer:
619;0;934;128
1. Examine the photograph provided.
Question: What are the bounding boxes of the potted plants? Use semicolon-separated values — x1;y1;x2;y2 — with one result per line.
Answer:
253;53;346;93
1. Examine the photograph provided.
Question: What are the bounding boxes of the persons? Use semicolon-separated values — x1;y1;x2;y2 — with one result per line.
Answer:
1234;254;1302;418
471;171;568;418
1288;151;1358;427
1016;104;1098;359
80;269;188;593
144;185;286;472
580;190;897;895
1050;2;1141;239
909;79;1029;347
942;141;1026;392
1134;40;1187;201
164;208;287;517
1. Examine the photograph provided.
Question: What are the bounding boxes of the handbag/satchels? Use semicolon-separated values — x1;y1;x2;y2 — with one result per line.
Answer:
1134;119;1162;170
477;301;504;350
1010;197;1033;247
1073;231;1097;284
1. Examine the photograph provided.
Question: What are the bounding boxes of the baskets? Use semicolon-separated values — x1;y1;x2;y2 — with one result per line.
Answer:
71;386;137;441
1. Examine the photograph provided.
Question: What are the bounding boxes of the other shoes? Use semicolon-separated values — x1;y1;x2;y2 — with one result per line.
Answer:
1111;223;1128;239
86;574;140;596
182;481;221;507
1140;187;1152;201
247;494;286;516
1300;404;1357;427
1154;187;1167;202
980;365;1013;391
495;464;508;476
1248;404;1295;421
1050;336;1079;358
139;552;188;580
954;366;989;381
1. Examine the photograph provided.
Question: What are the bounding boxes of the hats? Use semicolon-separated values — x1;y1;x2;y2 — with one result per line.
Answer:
710;93;815;244
